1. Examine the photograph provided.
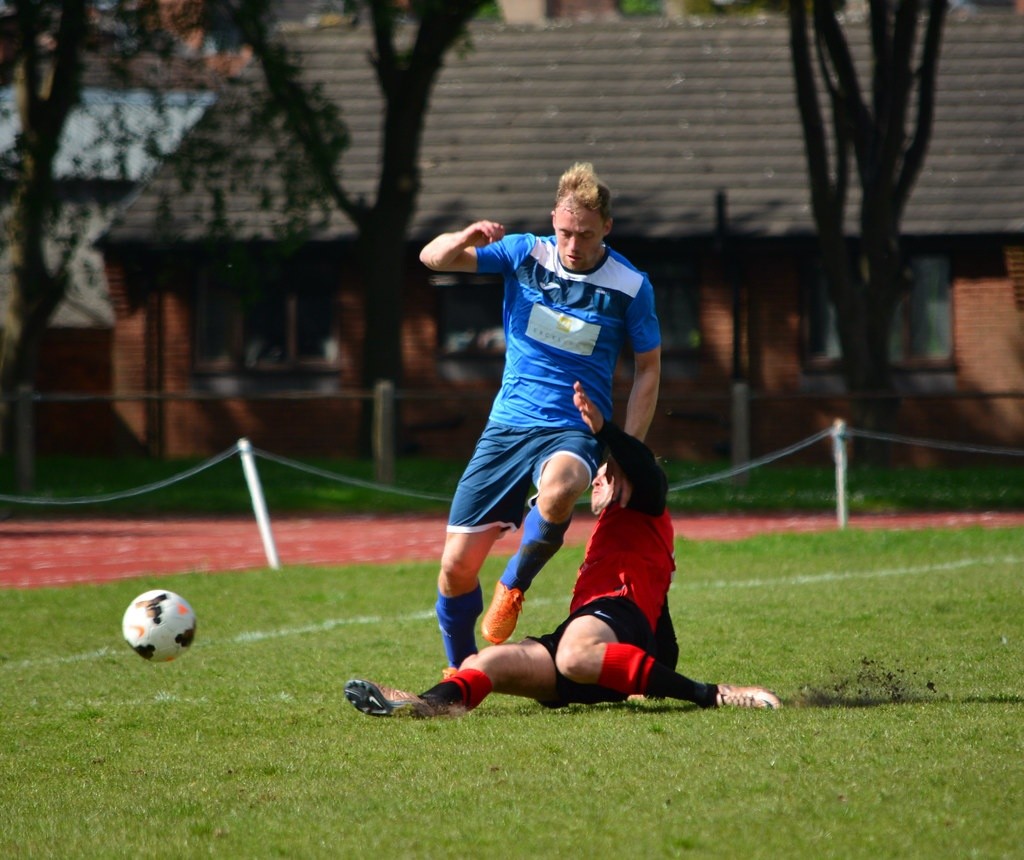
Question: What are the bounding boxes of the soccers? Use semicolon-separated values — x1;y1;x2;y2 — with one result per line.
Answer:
121;590;197;664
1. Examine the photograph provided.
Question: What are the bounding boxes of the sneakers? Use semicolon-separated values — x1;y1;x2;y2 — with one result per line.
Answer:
442;667;457;679
716;681;782;712
344;678;432;719
481;582;525;645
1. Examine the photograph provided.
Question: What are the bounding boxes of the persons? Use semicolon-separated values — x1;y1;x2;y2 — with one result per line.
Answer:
418;165;662;668
345;381;784;716
